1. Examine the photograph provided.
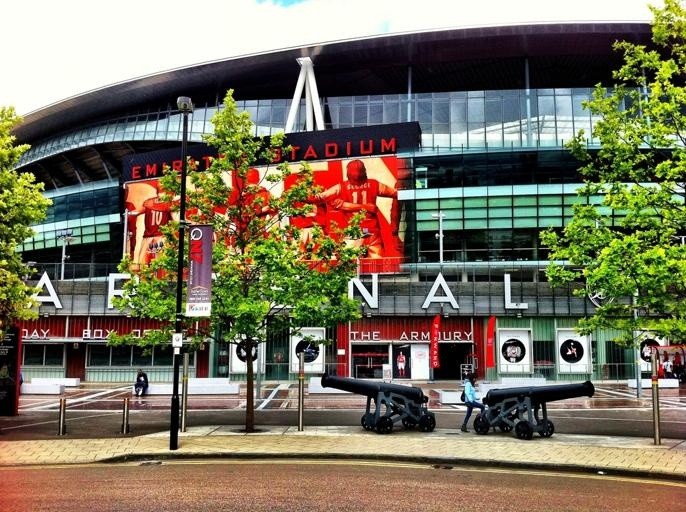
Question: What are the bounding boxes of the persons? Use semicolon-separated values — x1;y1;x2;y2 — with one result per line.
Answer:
243;168;273;236
396;350;407;376
253;171;327;245
299;160;401;260
133;367;148;396
458;371;485;431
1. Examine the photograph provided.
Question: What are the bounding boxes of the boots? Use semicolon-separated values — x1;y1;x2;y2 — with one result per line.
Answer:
461;423;470;433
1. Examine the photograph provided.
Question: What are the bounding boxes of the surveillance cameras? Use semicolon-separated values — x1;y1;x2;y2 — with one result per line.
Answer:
129;232;133;237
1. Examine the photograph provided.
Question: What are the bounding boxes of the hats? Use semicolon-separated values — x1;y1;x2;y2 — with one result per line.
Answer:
347;161;367;181
245;168;259;185
156;186;165;195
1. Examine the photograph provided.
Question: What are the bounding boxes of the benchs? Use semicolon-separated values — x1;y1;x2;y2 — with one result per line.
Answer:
188;378;230;383
307;377;547;395
132;383;241;395
31;377;80;386
20;384;65;394
628;378;679;389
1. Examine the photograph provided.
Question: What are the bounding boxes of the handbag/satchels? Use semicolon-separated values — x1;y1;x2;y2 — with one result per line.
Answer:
461;391;465;402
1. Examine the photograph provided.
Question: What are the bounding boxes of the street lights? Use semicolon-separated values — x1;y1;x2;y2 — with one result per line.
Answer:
170;93;194;451
